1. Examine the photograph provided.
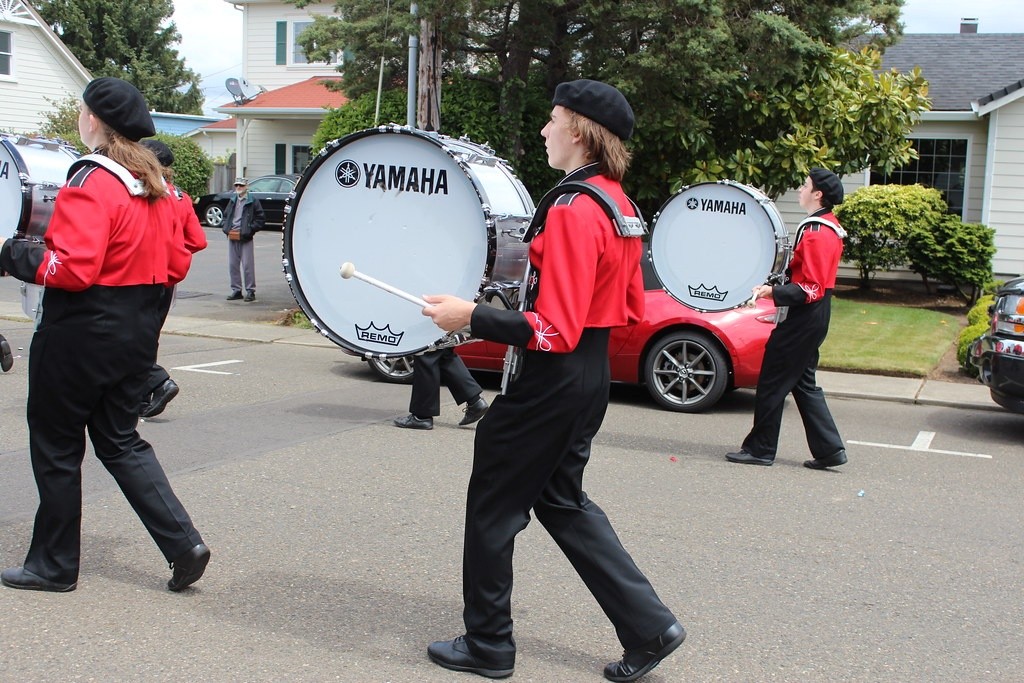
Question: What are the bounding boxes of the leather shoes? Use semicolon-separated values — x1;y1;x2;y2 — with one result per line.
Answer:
0;567;77;592
428;635;514;676
459;398;489;425
803;450;848;469
138;379;179;417
725;449;773;466
168;543;211;591
394;414;433;430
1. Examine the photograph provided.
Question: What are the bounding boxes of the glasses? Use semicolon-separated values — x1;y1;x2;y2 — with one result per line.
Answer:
234;184;245;187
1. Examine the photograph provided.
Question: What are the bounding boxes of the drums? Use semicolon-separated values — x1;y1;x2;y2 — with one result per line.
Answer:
647;178;789;315
0;134;79;278
279;123;536;360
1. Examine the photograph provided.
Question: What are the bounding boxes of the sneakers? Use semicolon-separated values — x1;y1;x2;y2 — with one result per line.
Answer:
604;621;686;682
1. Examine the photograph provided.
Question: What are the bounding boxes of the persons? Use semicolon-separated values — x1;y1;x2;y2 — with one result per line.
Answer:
0;334;13;372
424;83;686;683
222;178;264;302
395;350;489;428
138;141;207;417
726;166;849;468
0;78;211;592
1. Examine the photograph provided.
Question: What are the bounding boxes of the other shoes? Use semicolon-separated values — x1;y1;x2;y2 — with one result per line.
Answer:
0;341;13;372
227;291;243;300
244;292;255;302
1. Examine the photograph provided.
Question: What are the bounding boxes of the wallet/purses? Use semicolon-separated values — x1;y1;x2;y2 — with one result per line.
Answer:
229;230;240;240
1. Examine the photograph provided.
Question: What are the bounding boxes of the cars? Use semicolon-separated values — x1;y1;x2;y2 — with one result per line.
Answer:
342;236;775;413
967;276;1024;417
192;173;301;227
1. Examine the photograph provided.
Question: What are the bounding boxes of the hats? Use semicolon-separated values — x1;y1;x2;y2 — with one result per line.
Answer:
83;77;156;142
552;79;634;141
809;168;843;206
234;178;248;185
140;140;175;167
